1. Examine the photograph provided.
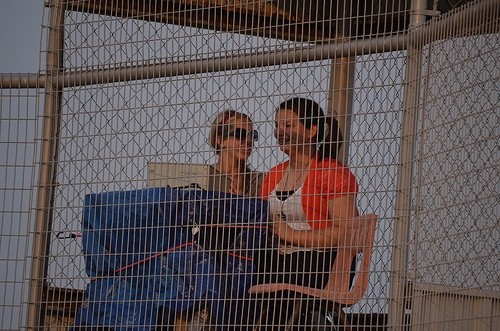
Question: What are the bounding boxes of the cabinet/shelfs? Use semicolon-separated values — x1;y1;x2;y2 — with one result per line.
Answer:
404;280;500;331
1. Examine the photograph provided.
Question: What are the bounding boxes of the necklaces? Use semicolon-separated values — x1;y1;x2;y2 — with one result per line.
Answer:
277;152;315;220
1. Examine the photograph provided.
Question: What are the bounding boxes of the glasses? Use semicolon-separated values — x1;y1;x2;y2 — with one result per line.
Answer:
219;127;259;142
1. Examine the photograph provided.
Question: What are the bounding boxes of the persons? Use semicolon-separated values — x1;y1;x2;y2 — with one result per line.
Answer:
258;94;360;331
209;108;269;195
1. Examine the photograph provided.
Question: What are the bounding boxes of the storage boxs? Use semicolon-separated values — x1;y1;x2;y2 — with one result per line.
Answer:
146;160;235;192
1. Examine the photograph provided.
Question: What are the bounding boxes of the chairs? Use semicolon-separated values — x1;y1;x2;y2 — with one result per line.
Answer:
248;213;380;331
